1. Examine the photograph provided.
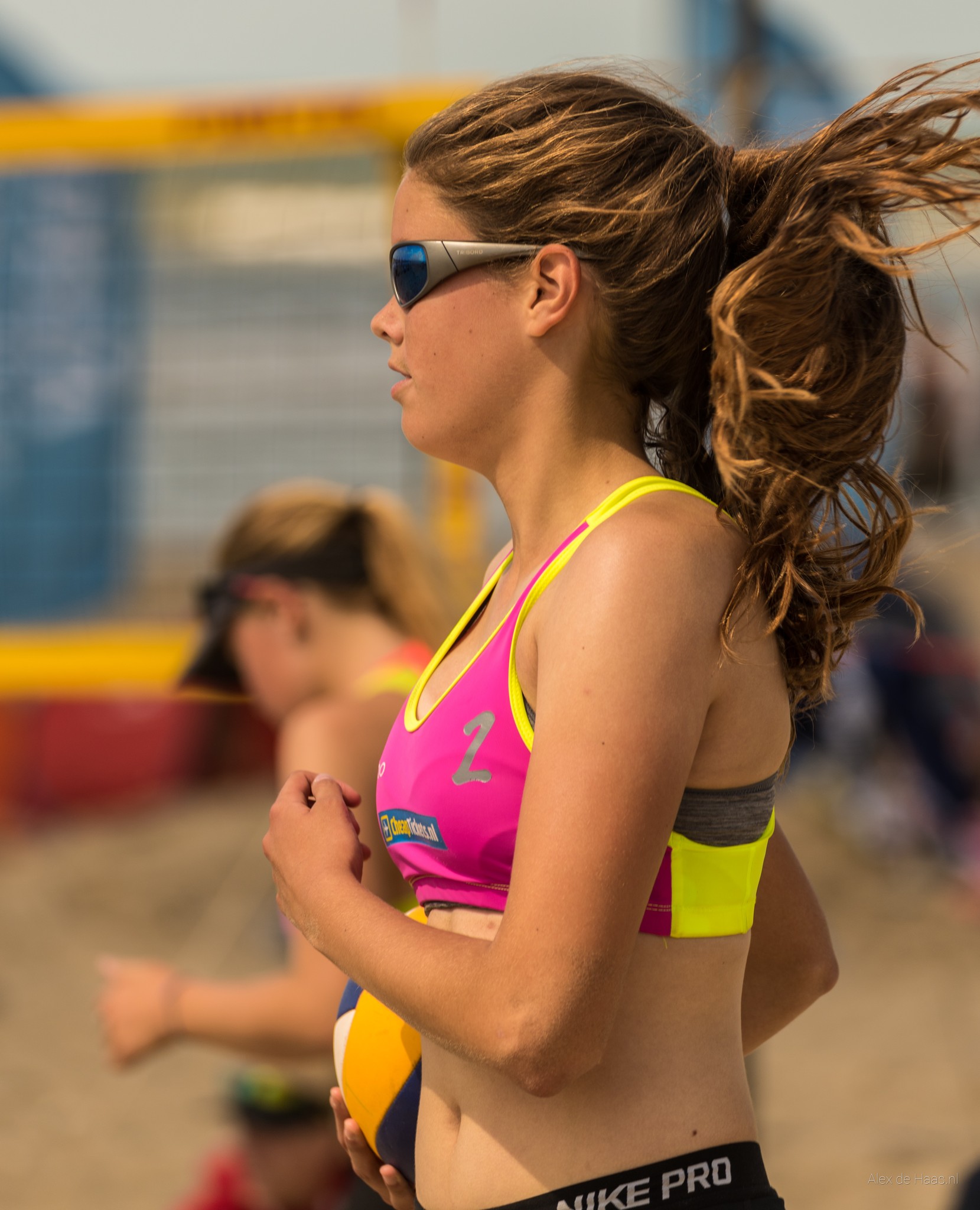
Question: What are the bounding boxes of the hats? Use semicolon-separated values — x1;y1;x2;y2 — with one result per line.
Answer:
176;548;370;695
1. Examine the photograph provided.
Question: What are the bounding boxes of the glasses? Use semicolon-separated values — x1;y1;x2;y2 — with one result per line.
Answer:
190;580;276;619
388;237;544;312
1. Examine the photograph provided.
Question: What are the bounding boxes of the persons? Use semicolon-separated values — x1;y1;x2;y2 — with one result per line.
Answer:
103;478;453;1209
260;55;980;1210
